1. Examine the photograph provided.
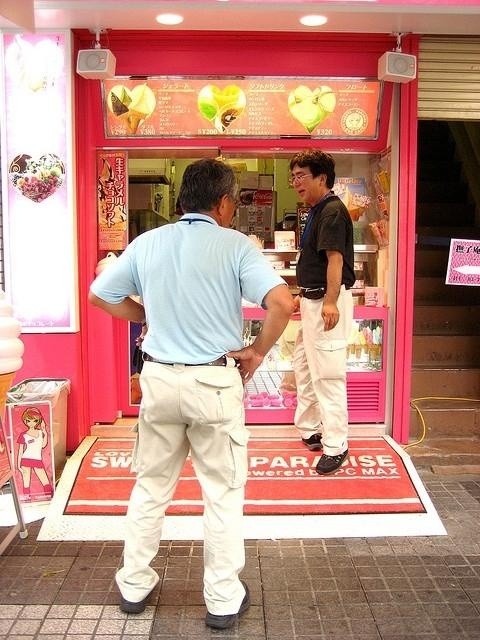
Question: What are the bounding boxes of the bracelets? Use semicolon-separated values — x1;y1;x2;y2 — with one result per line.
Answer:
141;320;147;327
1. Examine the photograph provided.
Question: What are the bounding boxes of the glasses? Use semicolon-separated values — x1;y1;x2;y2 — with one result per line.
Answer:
288;174;311;187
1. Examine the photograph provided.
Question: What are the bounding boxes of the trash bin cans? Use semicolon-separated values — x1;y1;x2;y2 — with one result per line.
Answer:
5;377;70;486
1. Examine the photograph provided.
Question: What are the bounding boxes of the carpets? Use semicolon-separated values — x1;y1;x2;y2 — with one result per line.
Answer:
60;439;426;515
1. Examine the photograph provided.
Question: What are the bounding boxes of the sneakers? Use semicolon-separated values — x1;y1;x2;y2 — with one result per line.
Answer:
121;589;152;614
301;434;323;450
206;581;249;629
315;448;349;475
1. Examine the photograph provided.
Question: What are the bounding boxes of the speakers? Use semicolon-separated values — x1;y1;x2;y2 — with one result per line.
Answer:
76;48;116;79
377;52;416;84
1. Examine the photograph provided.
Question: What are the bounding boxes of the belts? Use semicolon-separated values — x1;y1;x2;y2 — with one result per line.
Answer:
298;284;350;300
142;352;226;366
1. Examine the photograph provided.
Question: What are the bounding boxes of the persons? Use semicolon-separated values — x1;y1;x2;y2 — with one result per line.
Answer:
15;407;54;499
288;145;357;477
85;157;293;631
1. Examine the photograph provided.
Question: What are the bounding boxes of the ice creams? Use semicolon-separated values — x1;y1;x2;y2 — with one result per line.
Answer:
0;288;25;421
